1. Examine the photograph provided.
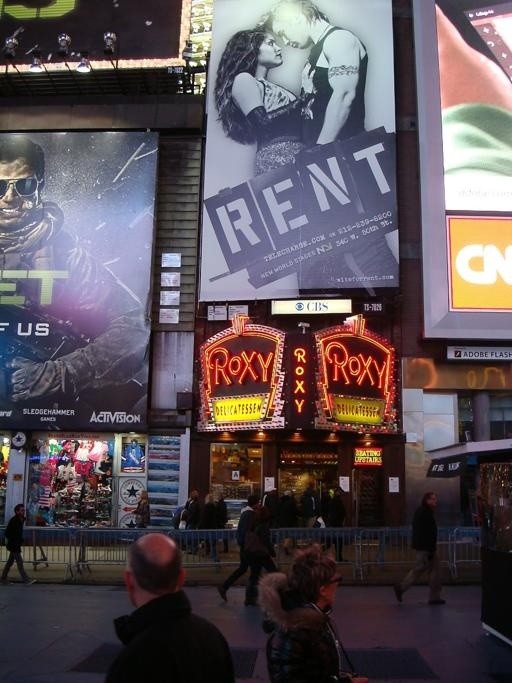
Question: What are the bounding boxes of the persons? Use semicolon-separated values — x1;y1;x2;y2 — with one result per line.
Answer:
0;132;152;423
1;501;37;587
246;503;279;605
269;0;395;286
263;544;374;683
216;492;261;603
127;488;351;563
222;24;307;180
101;524;238;683
390;490;448;606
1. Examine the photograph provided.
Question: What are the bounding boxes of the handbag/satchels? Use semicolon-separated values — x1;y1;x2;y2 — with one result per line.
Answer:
179;520;186;529
246;532;267;552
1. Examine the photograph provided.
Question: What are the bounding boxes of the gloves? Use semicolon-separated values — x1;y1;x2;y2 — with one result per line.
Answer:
12;355;75;402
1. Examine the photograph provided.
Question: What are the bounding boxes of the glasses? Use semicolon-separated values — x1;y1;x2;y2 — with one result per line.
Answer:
331;576;343;584
0;178;45;198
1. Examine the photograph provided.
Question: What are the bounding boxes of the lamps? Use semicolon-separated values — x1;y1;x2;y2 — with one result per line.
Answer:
5;30;121;73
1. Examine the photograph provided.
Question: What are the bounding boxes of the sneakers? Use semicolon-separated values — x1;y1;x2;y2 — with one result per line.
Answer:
216;585;227;602
1;579;14;585
26;580;36;585
394;584;402;601
245;599;259;607
429;599;446;606
